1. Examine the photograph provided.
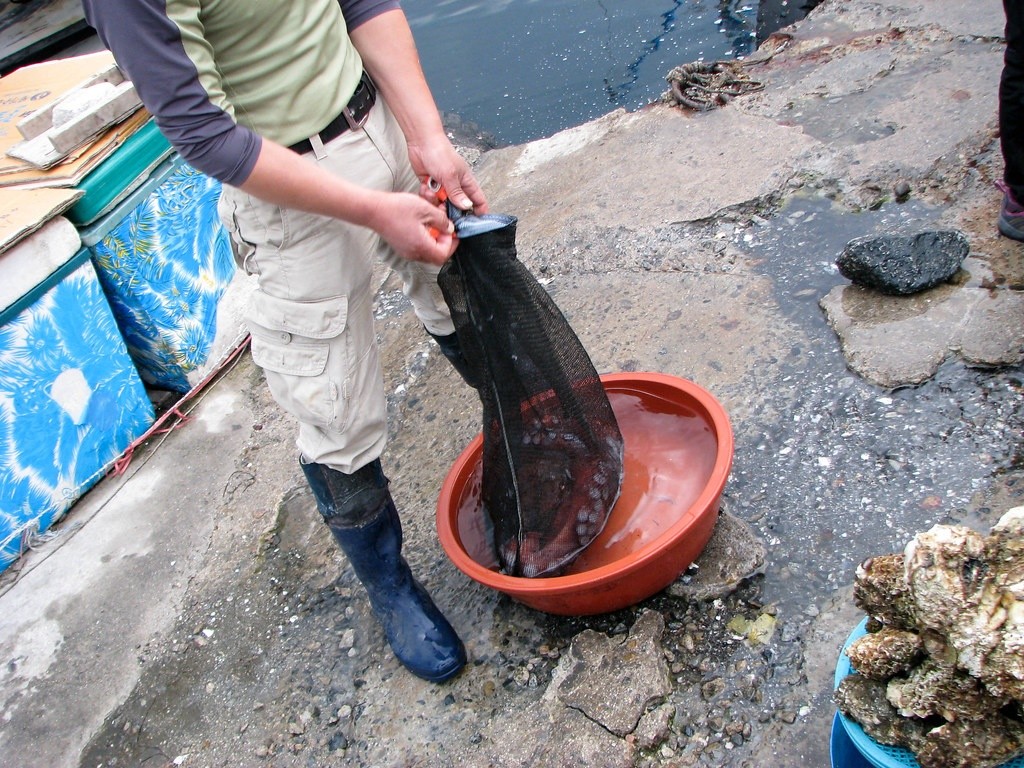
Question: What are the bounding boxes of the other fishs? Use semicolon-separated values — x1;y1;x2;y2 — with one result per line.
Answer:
504;414;616;578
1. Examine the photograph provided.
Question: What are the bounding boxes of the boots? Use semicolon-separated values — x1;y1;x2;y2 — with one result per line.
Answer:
298;452;467;684
422;323;521;404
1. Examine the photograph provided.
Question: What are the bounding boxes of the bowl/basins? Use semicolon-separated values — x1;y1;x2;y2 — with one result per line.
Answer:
432;370;740;622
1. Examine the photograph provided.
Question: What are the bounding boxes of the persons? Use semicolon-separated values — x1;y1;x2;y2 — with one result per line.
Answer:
82;0;488;683
998;0;1024;243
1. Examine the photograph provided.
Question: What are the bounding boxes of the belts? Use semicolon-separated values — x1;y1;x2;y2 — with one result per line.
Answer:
287;64;378;156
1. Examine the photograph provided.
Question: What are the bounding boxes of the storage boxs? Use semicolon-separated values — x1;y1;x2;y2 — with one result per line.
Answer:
0;215;159;577
0;50;249;397
